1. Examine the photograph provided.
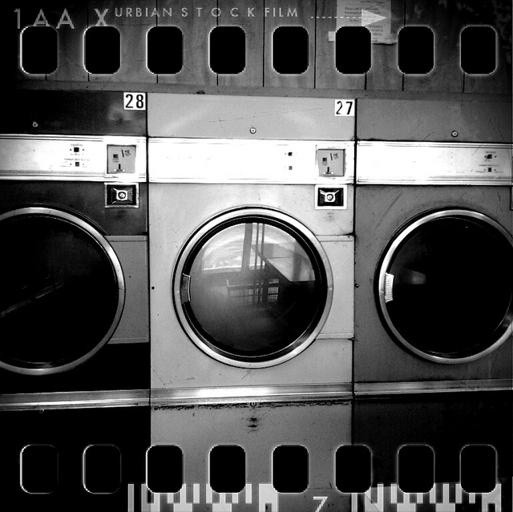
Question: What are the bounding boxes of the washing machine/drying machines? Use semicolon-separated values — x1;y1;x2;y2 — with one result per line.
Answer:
1;91;149;390
146;93;355;388
356;101;513;390
